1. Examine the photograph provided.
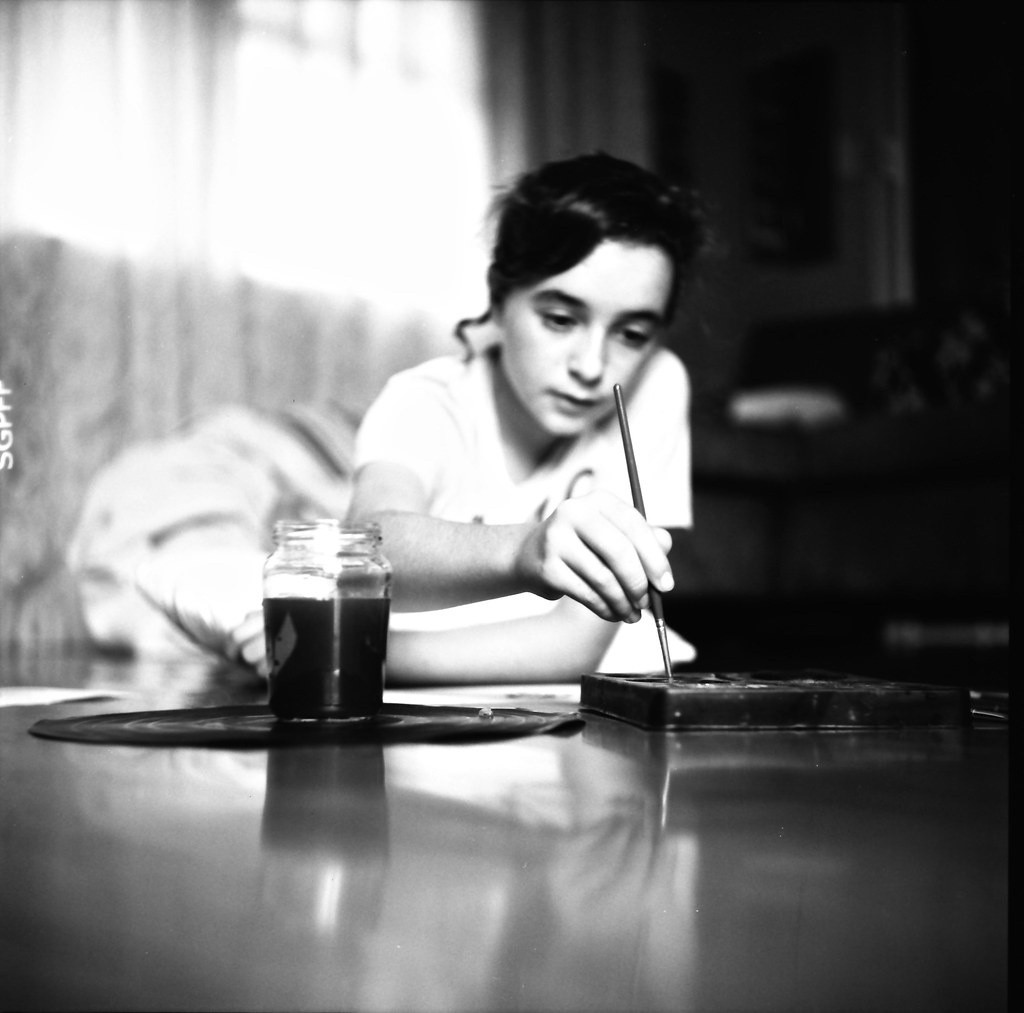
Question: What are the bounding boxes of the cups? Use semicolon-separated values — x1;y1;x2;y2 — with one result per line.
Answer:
261;519;393;721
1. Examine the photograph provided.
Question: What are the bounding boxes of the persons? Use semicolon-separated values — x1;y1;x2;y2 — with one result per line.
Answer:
230;154;697;684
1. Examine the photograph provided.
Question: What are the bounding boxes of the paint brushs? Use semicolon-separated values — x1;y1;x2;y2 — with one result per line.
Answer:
613;381;676;680
